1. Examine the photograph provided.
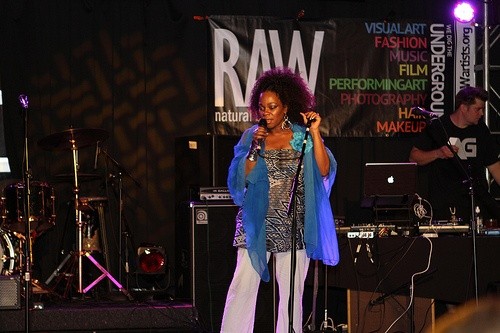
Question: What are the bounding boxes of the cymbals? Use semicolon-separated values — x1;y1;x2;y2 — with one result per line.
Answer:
37;129;110;151
56;173;101;182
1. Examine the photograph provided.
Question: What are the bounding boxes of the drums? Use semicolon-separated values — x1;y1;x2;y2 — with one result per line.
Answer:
0;182;56;224
80;197;109;252
0;227;25;276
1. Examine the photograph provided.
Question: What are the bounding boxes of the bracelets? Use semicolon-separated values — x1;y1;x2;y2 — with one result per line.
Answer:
246;140;258;162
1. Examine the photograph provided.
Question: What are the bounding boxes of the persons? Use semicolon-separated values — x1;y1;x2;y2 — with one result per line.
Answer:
409;87;500;219
219;68;340;333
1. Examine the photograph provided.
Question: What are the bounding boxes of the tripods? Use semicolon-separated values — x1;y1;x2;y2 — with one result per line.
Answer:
41;139;137;306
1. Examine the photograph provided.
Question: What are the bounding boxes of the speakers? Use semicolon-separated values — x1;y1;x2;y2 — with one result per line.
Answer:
190;201;316;330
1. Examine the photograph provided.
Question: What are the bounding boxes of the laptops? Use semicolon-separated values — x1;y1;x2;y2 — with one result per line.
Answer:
363;162;420;208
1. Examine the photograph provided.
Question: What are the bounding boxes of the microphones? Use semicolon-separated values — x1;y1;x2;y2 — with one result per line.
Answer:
16;91;29;109
257;119;267;150
414;107;438;119
